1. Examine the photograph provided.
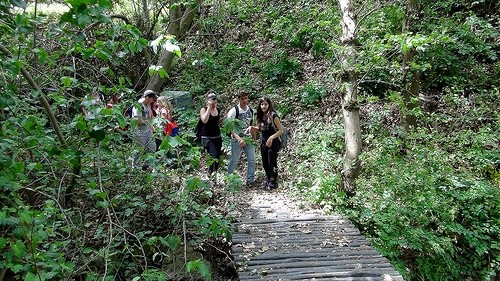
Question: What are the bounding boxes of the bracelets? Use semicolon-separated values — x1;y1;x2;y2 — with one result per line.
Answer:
238;138;241;143
251;126;253;130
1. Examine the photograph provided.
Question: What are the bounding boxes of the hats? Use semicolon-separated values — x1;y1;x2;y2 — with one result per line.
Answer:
144;90;158;99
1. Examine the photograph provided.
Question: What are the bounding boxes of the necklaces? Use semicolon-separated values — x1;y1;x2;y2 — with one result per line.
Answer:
211;109;217;116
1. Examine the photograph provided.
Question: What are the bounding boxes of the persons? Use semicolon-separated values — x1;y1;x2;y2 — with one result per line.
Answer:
81;85;172;175
200;91;225;186
225;89;257;190
244;97;284;192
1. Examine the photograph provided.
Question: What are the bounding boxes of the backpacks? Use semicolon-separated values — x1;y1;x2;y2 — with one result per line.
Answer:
123;105;144;129
226;105;253;137
194;120;202;146
160;108;179;137
271;112;287;147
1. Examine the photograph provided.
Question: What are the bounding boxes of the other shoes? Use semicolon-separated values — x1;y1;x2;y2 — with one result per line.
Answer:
265;183;278;191
246;180;261;187
259;181;270;190
